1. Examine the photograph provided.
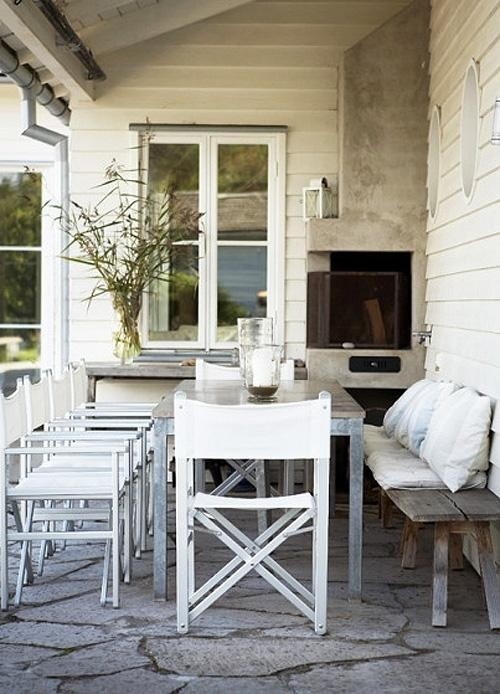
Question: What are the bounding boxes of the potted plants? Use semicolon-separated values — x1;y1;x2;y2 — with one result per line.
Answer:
20;115;207;365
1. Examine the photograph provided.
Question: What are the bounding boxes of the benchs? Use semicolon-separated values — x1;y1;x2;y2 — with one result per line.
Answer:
362;424;500;631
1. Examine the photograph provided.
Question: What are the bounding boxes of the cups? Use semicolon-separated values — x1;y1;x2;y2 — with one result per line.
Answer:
236;317;276;380
241;343;284;396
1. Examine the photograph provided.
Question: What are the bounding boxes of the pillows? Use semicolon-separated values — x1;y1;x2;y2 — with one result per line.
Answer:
382;379;492;493
